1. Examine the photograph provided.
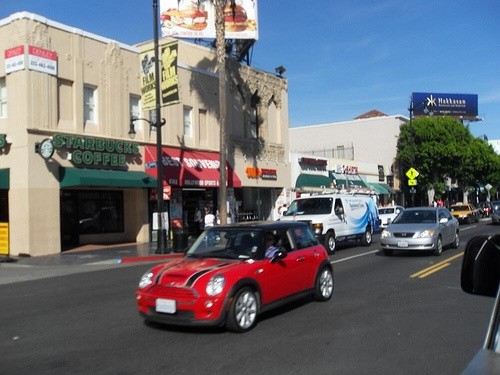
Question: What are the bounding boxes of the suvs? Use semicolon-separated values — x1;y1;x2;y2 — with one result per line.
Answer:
132;219;335;336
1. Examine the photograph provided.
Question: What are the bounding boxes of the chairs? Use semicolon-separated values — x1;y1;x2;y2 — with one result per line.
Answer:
240;234;254;247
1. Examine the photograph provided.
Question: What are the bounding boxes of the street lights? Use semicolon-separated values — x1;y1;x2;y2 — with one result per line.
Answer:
128;0;171;255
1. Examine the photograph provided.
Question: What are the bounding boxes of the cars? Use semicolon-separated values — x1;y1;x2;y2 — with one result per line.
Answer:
476;200;500;225
449;232;500;375
377;205;406;230
379;206;461;257
448;201;480;225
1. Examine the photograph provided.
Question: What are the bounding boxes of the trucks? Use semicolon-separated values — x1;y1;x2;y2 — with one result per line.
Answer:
282;185;380;254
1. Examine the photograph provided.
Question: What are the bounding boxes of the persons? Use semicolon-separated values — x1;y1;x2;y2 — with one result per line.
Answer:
192;206;216;239
265;232;280;257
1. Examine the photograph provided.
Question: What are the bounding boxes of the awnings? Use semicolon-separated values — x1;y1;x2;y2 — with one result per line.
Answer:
329;172;370;193
57;167;157;189
144;144;241;187
365;182;397;196
295;173;333;194
